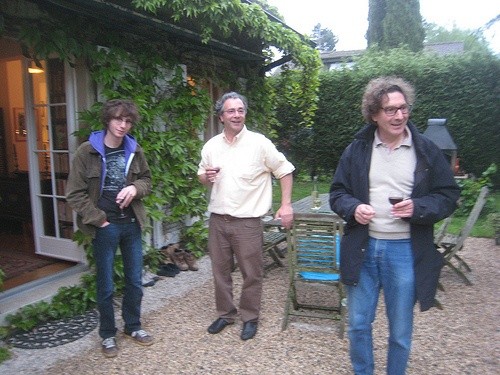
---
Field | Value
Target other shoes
[207,316,235,334]
[241,321,257,339]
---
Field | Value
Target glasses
[223,108,247,116]
[112,116,136,128]
[379,104,413,116]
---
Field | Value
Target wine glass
[116,193,127,218]
[387,190,405,219]
[209,161,220,182]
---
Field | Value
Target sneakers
[123,329,155,346]
[102,336,118,357]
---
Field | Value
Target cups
[310,190,321,211]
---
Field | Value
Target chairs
[230,205,288,277]
[280,219,346,340]
[431,186,488,283]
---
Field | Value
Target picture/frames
[13,107,41,142]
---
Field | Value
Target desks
[288,191,343,264]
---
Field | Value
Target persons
[66,99,153,356]
[329,76,459,375]
[197,92,296,339]
